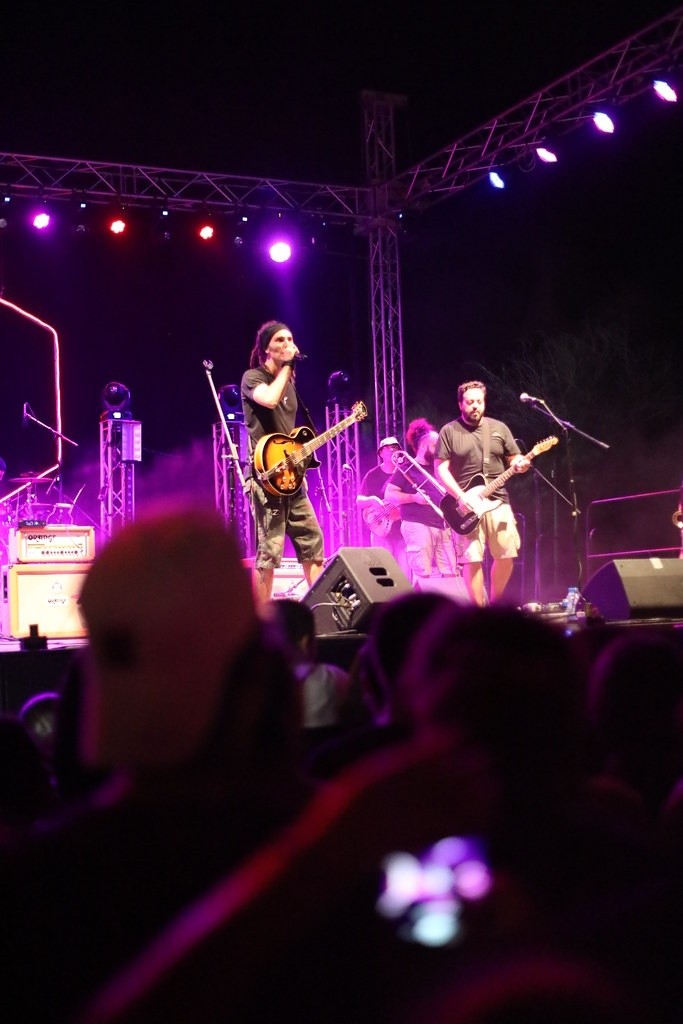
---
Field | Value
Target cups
[528,603,541,612]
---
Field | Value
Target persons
[384,417,460,581]
[434,381,532,606]
[241,321,327,607]
[1,512,683,1024]
[353,437,412,591]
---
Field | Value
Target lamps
[100,381,132,420]
[488,168,514,190]
[328,371,351,404]
[536,140,564,163]
[593,94,624,133]
[217,384,243,421]
[653,66,681,104]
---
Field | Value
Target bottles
[567,588,579,614]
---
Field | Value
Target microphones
[46,476,59,496]
[294,352,306,362]
[520,393,539,403]
[22,404,29,427]
[343,464,352,470]
[274,592,296,597]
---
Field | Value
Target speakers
[0,564,93,640]
[297,546,412,637]
[270,558,327,601]
[575,557,683,622]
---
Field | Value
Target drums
[0,499,72,566]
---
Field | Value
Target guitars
[363,499,402,538]
[253,400,368,497]
[439,435,559,535]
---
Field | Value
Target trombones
[391,450,447,520]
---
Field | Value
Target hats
[377,437,402,459]
[77,512,260,769]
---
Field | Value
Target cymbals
[9,477,53,484]
